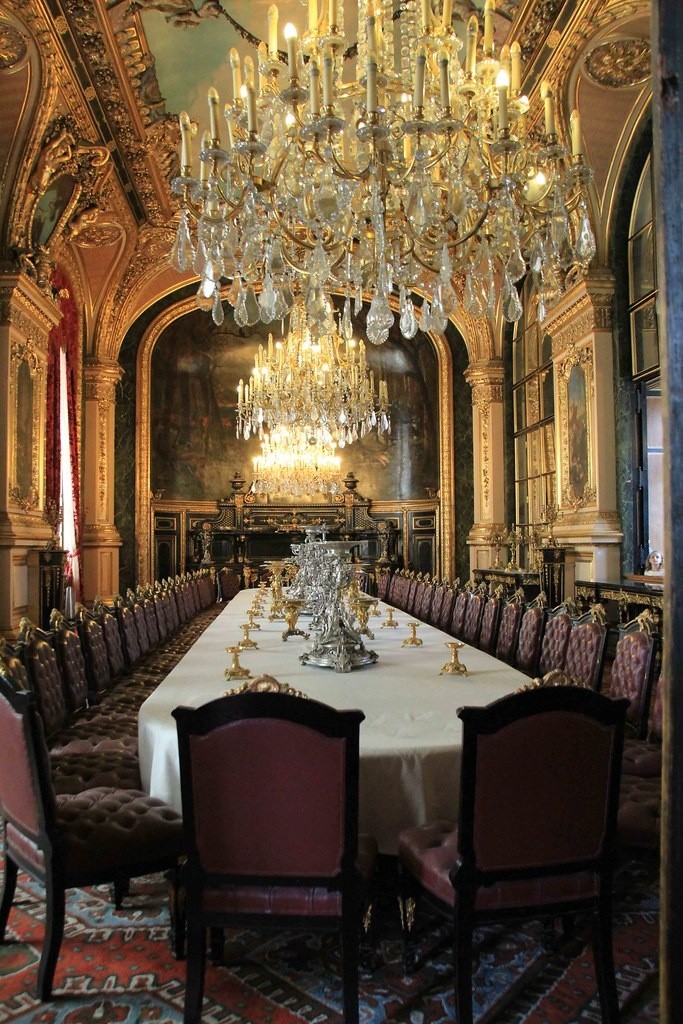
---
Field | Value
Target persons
[644,550,664,586]
[69,203,99,243]
[31,128,72,196]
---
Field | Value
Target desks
[139,587,532,880]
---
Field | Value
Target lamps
[234,291,391,450]
[250,417,343,498]
[170,0,597,346]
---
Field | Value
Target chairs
[0,566,665,1024]
